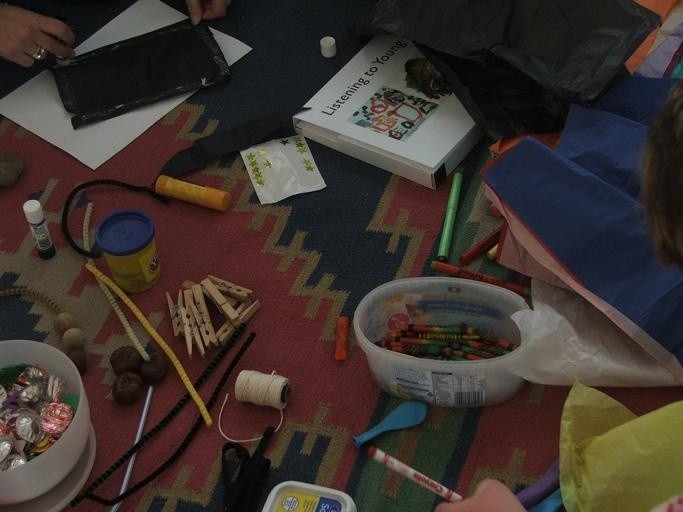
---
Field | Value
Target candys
[0,362,77,471]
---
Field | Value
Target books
[290,32,486,191]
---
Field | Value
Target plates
[0,421,97,512]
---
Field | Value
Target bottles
[92,207,161,297]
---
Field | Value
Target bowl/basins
[0,339,93,506]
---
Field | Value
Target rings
[34,46,47,62]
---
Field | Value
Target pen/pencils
[367,448,465,503]
[430,172,532,298]
[382,324,512,360]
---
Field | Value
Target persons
[0,0,230,72]
[432,76,682,511]
